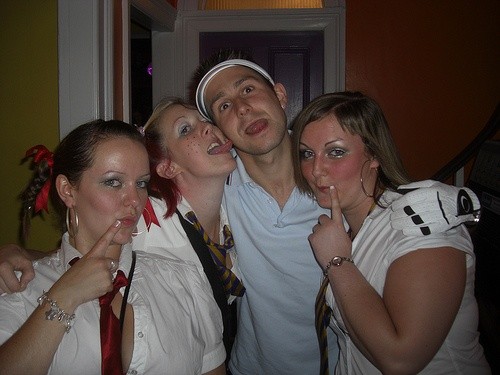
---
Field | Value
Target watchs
[323,256,354,277]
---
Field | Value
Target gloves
[390,179,481,237]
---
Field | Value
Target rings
[109,258,119,272]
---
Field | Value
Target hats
[196,59,275,122]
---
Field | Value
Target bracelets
[37,289,76,334]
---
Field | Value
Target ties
[311,192,384,375]
[69,257,129,375]
[185,211,246,298]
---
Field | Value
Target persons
[0,96,246,375]
[0,119,227,375]
[291,91,491,375]
[185,46,481,375]
